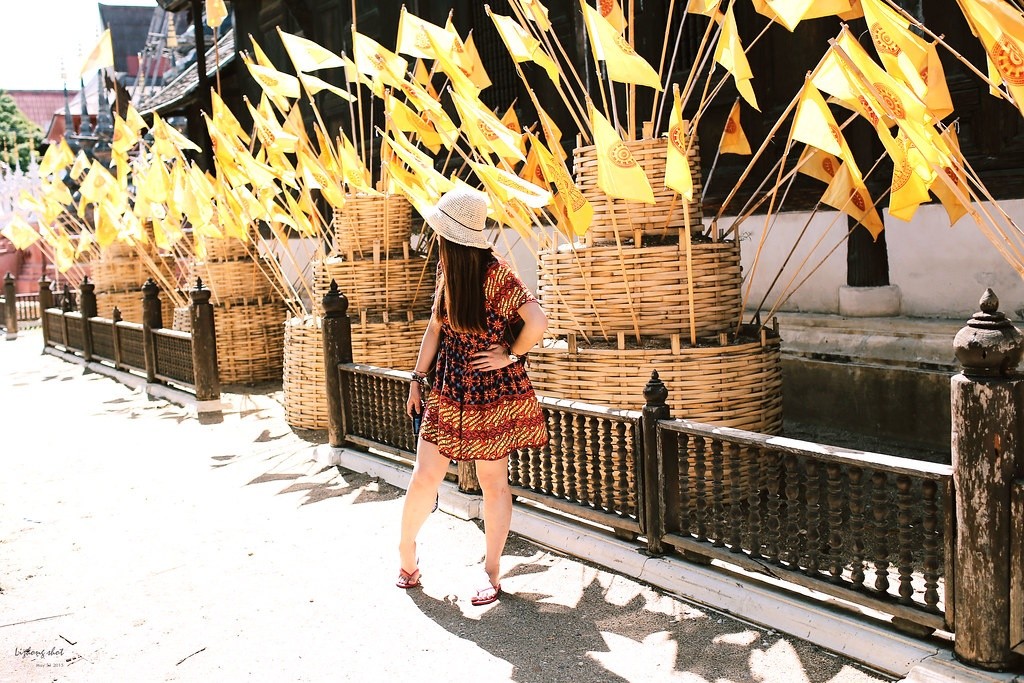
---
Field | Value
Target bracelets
[410,369,427,385]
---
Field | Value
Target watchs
[507,347,528,362]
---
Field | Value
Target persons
[394,184,548,605]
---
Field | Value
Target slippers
[471,566,501,604]
[397,555,420,588]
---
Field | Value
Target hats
[419,188,492,249]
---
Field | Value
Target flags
[0,0,1024,341]
